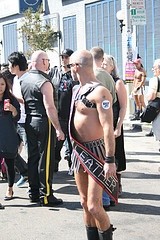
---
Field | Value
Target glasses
[67,64,78,68]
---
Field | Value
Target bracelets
[105,156,115,163]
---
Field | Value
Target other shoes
[4,191,13,200]
[17,176,29,186]
[40,195,63,207]
[146,132,154,136]
[103,205,111,211]
[68,167,73,175]
[110,201,116,206]
[30,193,44,202]
[0,204,4,209]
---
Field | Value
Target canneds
[4,99,10,110]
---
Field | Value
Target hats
[59,49,73,57]
[133,59,141,64]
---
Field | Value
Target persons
[147,59,160,142]
[90,47,116,209]
[131,58,147,121]
[0,52,29,201]
[101,54,126,197]
[68,50,116,240]
[46,48,80,176]
[21,50,64,206]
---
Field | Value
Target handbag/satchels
[140,98,160,123]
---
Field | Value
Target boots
[86,226,99,240]
[131,110,140,120]
[98,224,113,240]
[116,173,122,196]
